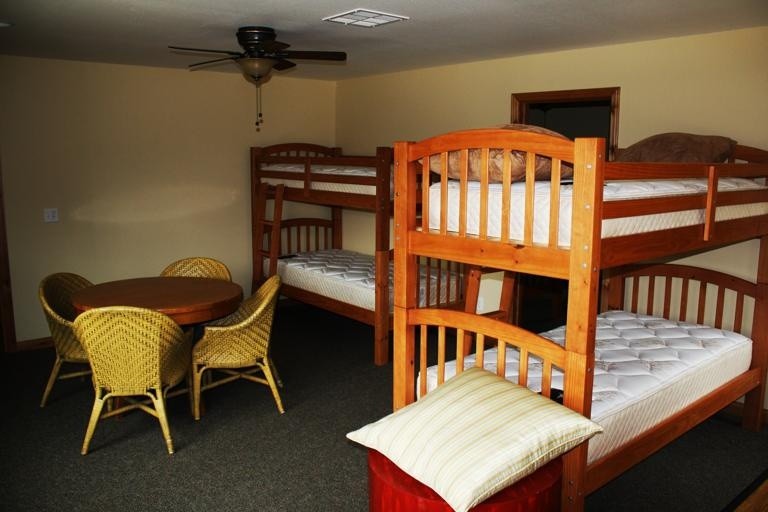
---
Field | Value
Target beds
[397,123,767,511]
[250,142,469,366]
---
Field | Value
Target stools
[368,446,562,512]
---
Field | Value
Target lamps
[236,57,280,133]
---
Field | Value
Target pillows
[609,132,738,163]
[345,365,604,511]
[417,123,574,184]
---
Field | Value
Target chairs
[192,274,286,421]
[72,305,194,455]
[161,257,243,386]
[39,272,114,413]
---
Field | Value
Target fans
[168,25,347,71]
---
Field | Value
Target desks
[69,276,243,420]
